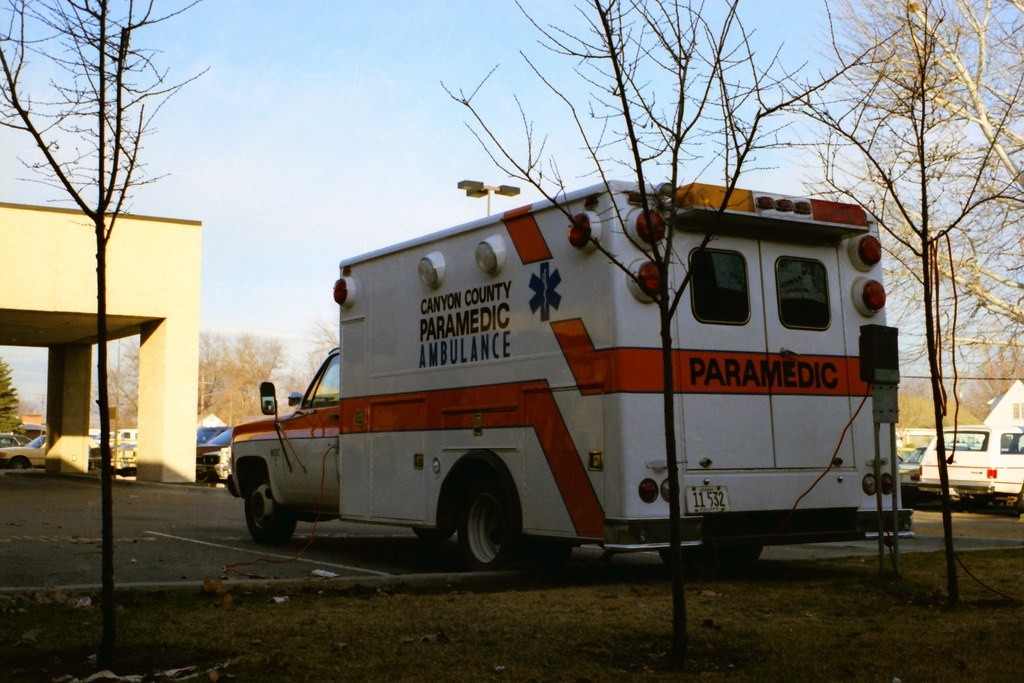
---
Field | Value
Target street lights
[458,179,521,215]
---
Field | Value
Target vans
[916,424,1024,515]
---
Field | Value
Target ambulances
[226,179,916,573]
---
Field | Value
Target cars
[0,424,234,485]
[898,442,981,511]
